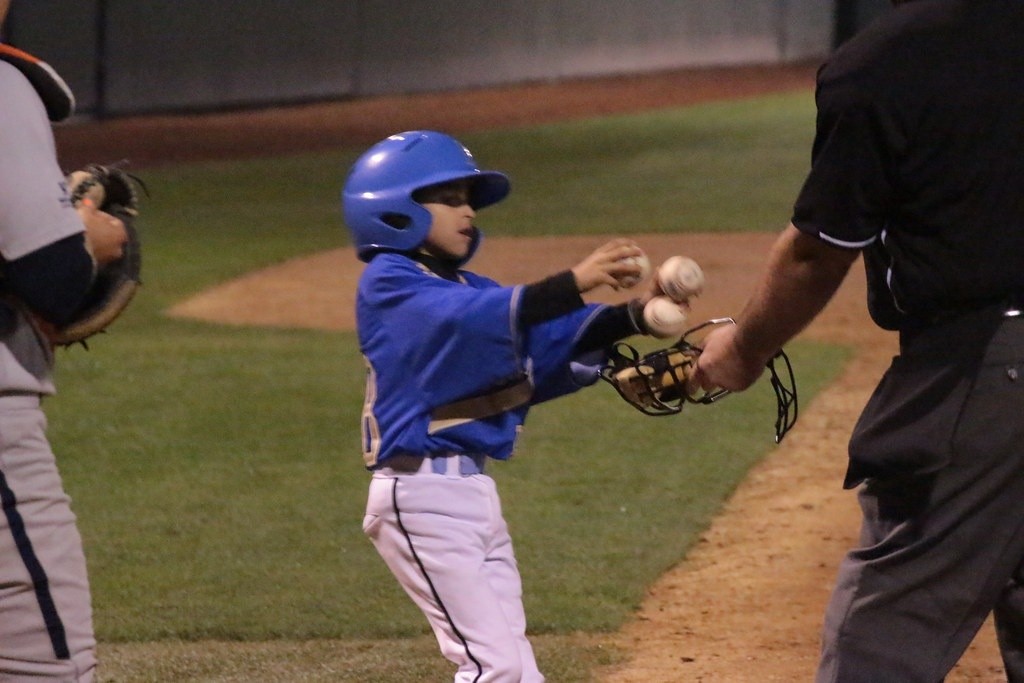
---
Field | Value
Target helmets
[340,129,512,268]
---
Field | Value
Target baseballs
[615,247,648,286]
[643,295,692,339]
[657,255,704,300]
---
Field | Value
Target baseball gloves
[40,160,142,348]
[612,314,740,416]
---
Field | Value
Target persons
[596,0,1024,683]
[338,130,692,683]
[0,0,142,681]
